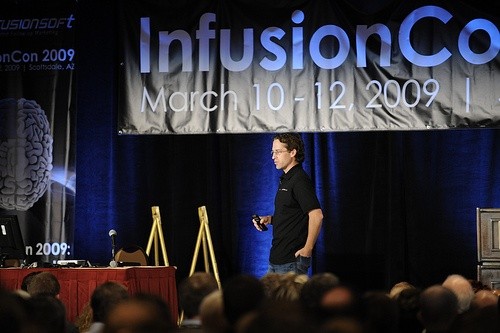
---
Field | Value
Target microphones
[109,261,140,267]
[109,230,117,257]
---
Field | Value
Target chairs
[114,246,150,266]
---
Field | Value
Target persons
[251,131,323,274]
[176,271,218,333]
[81,281,129,333]
[199,271,500,333]
[0,271,81,333]
[104,292,177,333]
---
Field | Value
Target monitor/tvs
[0,215,27,267]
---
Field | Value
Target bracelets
[267,216,269,224]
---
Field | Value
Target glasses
[270,148,289,155]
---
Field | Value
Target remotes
[252,215,268,231]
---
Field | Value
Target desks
[0,264,179,327]
